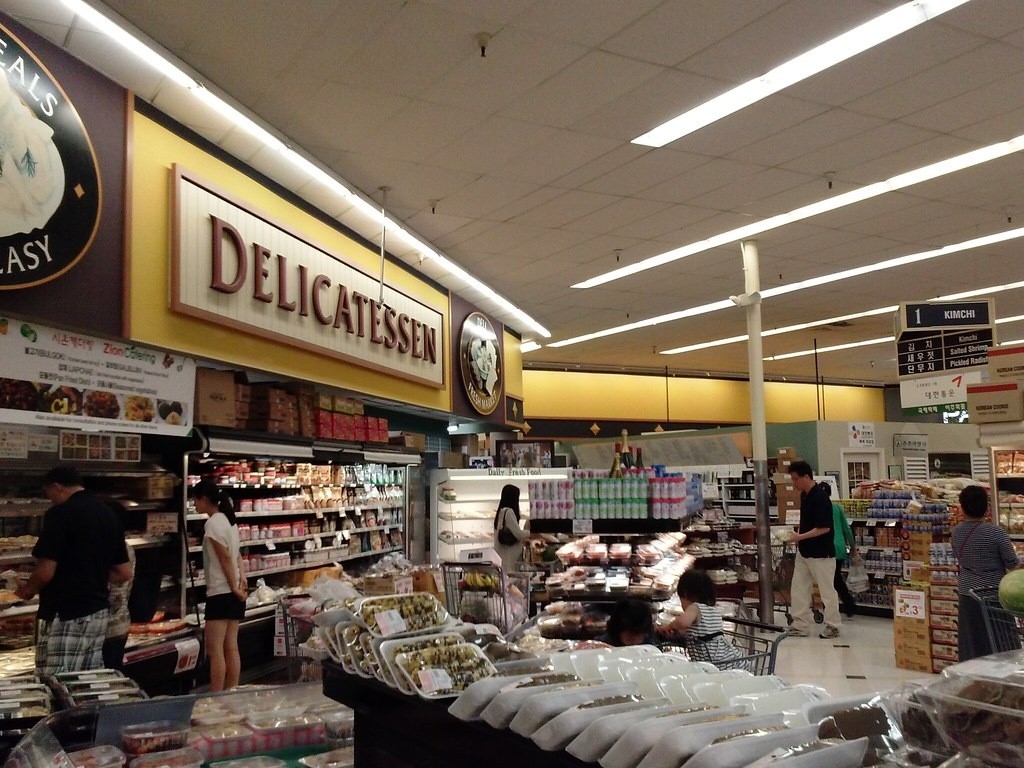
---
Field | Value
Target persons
[784,461,841,638]
[191,481,248,692]
[658,570,749,671]
[493,484,530,570]
[951,485,1021,664]
[819,482,858,620]
[101,502,136,670]
[14,466,133,677]
[598,601,686,648]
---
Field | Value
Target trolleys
[649,616,786,676]
[439,559,535,634]
[744,538,824,626]
[280,593,324,681]
[969,583,1024,654]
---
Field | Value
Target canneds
[234,498,305,573]
[528,478,687,519]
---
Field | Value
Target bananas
[466,569,485,587]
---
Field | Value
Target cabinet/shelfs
[187,425,422,694]
[682,526,760,599]
[430,468,573,567]
[665,463,779,518]
[841,519,902,620]
[989,446,1024,541]
[0,408,204,697]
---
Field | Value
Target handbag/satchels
[498,509,518,546]
[846,552,870,592]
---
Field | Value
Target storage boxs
[274,608,309,657]
[967,343,1024,424]
[875,528,897,547]
[289,567,342,587]
[784,560,824,610]
[364,571,446,605]
[773,448,804,524]
[194,368,426,453]
[893,520,961,674]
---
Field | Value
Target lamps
[60,0,551,340]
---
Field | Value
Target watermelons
[997,569,1024,614]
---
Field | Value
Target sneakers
[786,625,807,636]
[820,625,839,639]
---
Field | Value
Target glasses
[791,476,799,483]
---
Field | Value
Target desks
[529,592,674,617]
[323,659,601,768]
